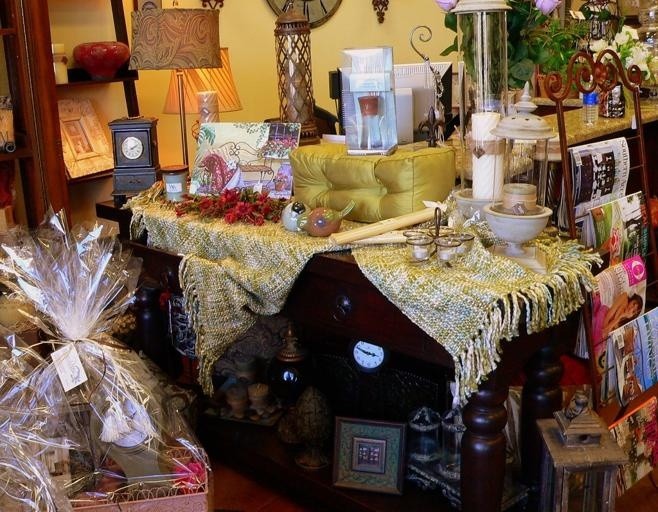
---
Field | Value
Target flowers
[587,29,648,82]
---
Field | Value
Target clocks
[109,121,161,192]
[90,389,175,498]
[353,341,385,371]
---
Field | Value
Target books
[189,122,302,200]
[556,137,658,498]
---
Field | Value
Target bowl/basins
[454,188,502,219]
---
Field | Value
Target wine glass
[483,202,554,258]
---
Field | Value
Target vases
[601,80,624,117]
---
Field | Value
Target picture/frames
[330,417,407,494]
[58,97,114,179]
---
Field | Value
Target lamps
[163,48,241,113]
[127,8,221,184]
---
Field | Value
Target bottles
[490,80,558,213]
[581,75,600,127]
[406,402,465,480]
[160,164,188,203]
[51,43,69,85]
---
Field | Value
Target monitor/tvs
[336,62,453,144]
